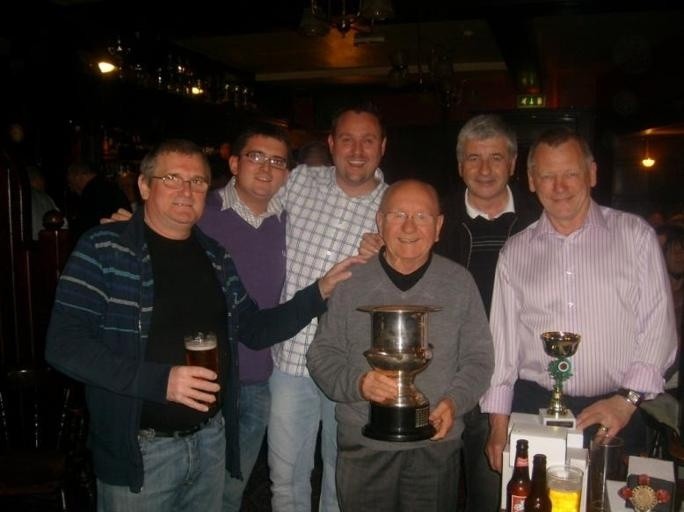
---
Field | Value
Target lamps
[302,0,393,38]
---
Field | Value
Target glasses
[238,150,289,171]
[147,174,211,194]
[379,210,435,227]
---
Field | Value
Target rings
[599,424,608,432]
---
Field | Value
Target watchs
[615,387,642,409]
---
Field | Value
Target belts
[140,418,215,439]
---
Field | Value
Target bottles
[507,440,531,512]
[524,454,552,512]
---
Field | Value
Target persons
[265,105,391,511]
[305,178,494,508]
[24,165,68,241]
[43,135,367,511]
[662,236,684,352]
[62,158,133,222]
[475,128,679,475]
[356,113,530,512]
[97,124,295,512]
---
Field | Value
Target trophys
[356,302,443,443]
[539,329,582,430]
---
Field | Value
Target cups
[184,331,220,408]
[590,436,624,512]
[546,465,584,512]
